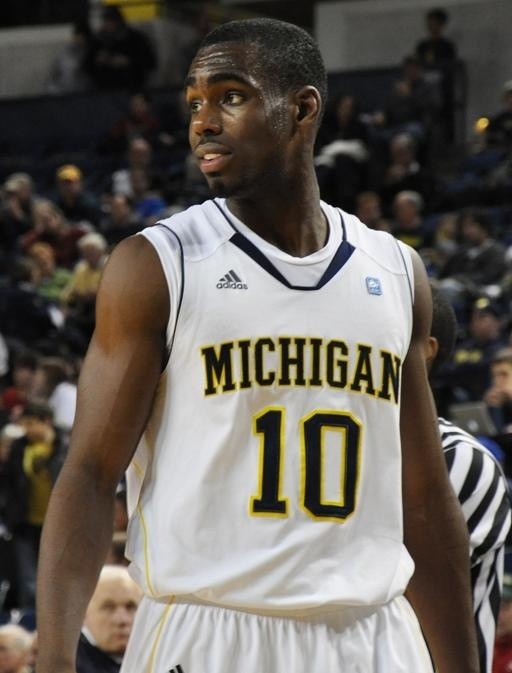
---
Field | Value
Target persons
[0,2,511,672]
